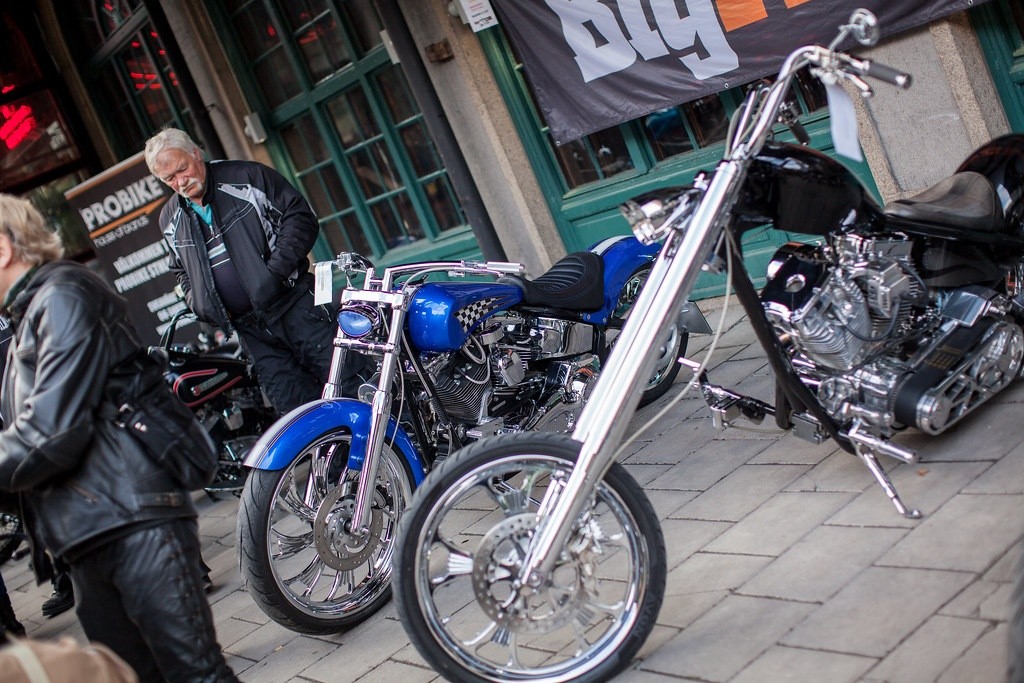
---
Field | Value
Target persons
[0,194,244,683]
[144,129,372,416]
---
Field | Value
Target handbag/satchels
[12,332,218,491]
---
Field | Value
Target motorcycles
[142,303,272,496]
[230,245,692,638]
[389,10,1024,683]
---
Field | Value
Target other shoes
[42,588,73,615]
[3,619,25,636]
[200,575,213,592]
